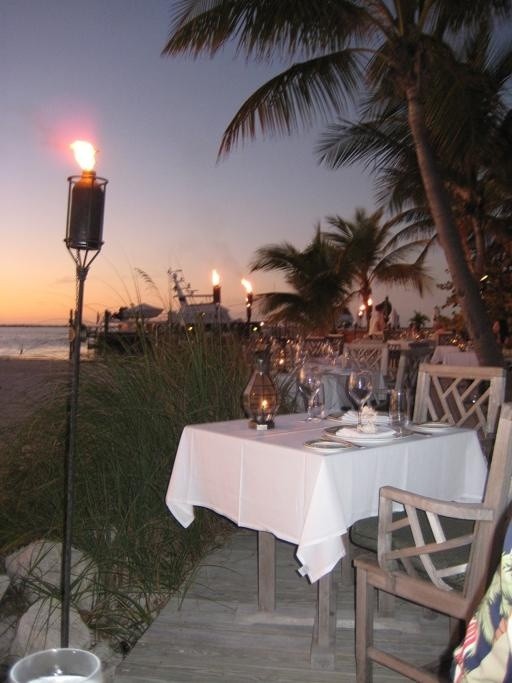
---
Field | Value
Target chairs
[253,333,507,462]
[350,403,512,683]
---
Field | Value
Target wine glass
[295,367,322,422]
[322,344,338,373]
[347,371,374,433]
[389,389,411,437]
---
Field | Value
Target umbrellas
[122,302,163,319]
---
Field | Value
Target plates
[417,420,456,430]
[323,424,403,444]
[303,440,352,451]
